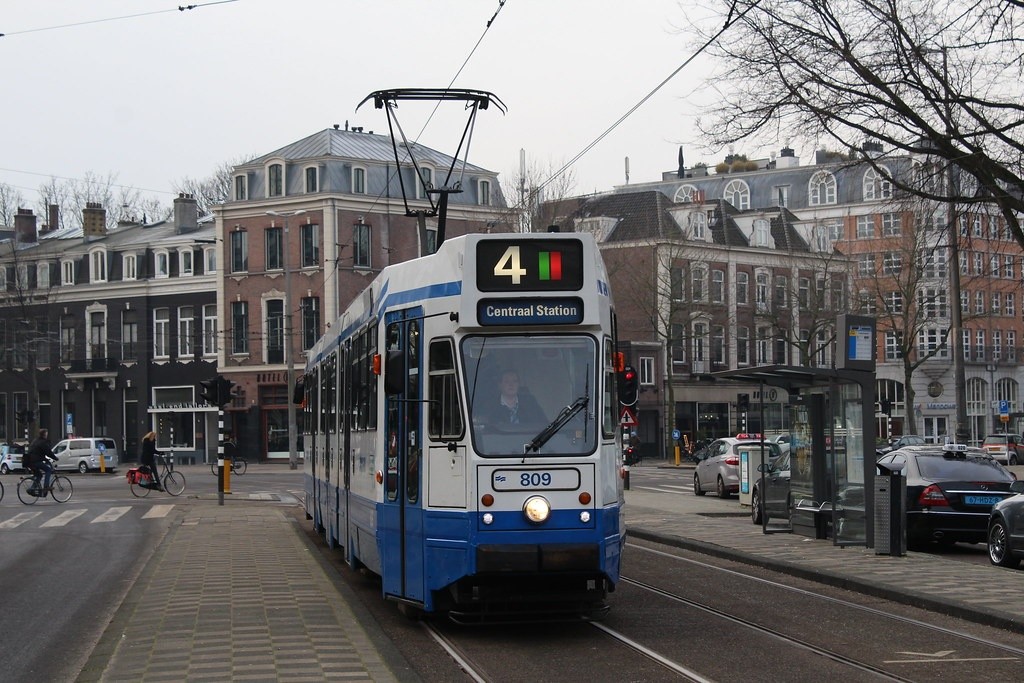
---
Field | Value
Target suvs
[875,434,926,459]
[981,433,1024,466]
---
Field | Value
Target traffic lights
[14,411,27,423]
[200,378,218,405]
[623,367,637,400]
[222,378,238,404]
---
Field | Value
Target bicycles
[212,445,247,476]
[129,454,187,499]
[17,459,73,506]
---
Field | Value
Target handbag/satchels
[22,454,31,467]
[127,467,140,484]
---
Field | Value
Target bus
[291,86,629,628]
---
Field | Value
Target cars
[0,444,31,474]
[751,447,883,525]
[839,444,1018,552]
[986,480,1024,567]
[693,432,782,499]
[765,432,808,457]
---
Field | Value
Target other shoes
[45,487,55,491]
[157,486,166,492]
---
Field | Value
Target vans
[39,437,118,473]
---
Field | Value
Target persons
[139,432,165,492]
[27,429,59,491]
[223,437,236,465]
[481,370,547,423]
[687,437,711,461]
[630,432,640,446]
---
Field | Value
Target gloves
[159,451,165,455]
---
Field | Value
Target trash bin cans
[874,462,906,556]
